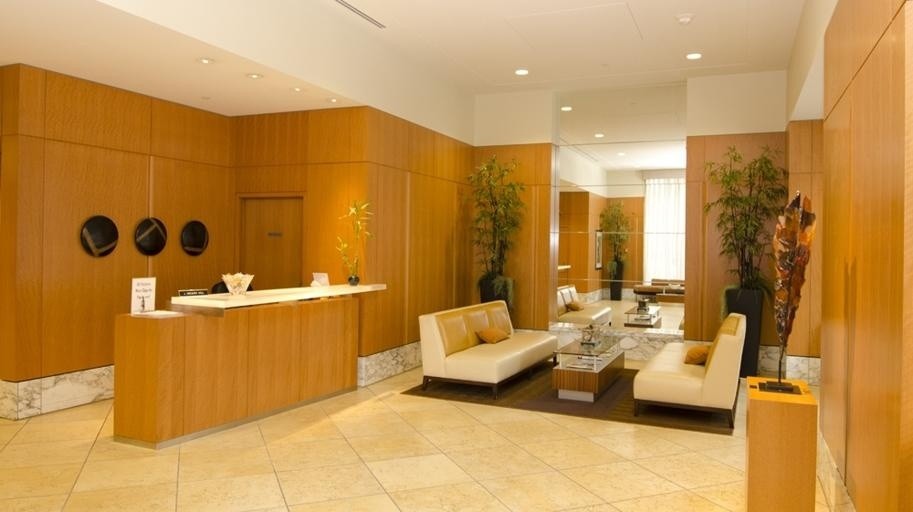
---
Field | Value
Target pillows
[685,344,709,365]
[475,328,510,343]
[566,300,583,310]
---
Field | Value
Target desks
[744,376,818,512]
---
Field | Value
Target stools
[633,286,663,302]
[665,286,685,295]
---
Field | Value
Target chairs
[632,313,747,428]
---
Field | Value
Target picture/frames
[595,230,603,271]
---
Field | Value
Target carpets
[401,361,741,436]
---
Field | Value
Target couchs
[417,299,558,399]
[556,284,613,327]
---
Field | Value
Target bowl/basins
[221,272,254,297]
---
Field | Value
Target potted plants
[600,200,632,300]
[465,155,526,310]
[336,202,375,286]
[704,141,787,378]
